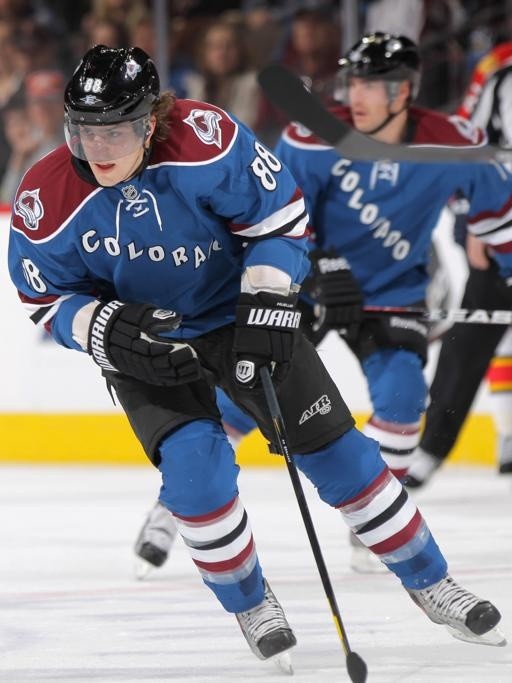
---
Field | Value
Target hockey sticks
[259,364,369,682]
[256,60,510,161]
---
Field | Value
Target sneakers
[132,499,179,570]
[230,577,298,661]
[406,572,502,637]
[395,447,444,489]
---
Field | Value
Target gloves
[86,300,204,392]
[227,290,295,396]
[309,250,370,345]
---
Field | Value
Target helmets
[341,30,423,104]
[60,40,160,127]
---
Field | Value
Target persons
[5,40,502,661]
[131,28,512,571]
[398,38,511,489]
